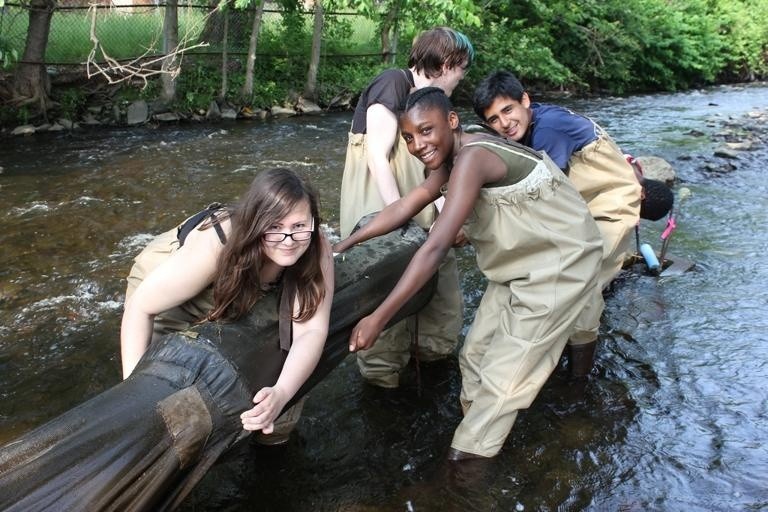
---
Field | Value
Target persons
[331,86,604,459]
[121,167,334,446]
[339,26,474,389]
[617,147,673,221]
[472,71,642,377]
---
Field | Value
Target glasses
[261,216,316,242]
